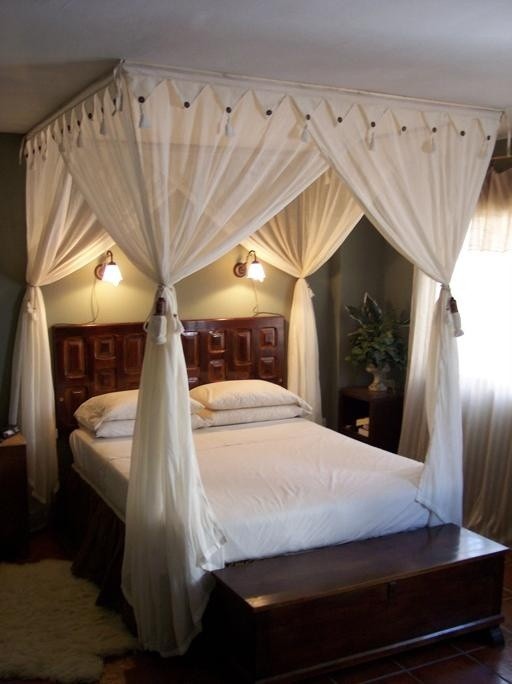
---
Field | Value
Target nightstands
[332,382,405,454]
[1,426,29,562]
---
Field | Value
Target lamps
[231,248,267,281]
[92,248,124,288]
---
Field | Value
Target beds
[48,314,465,646]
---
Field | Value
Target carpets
[0,547,138,681]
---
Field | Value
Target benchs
[203,519,510,679]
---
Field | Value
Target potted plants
[338,287,414,393]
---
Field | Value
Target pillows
[187,378,316,413]
[194,401,313,427]
[73,410,214,438]
[69,384,206,431]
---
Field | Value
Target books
[355,416,369,437]
